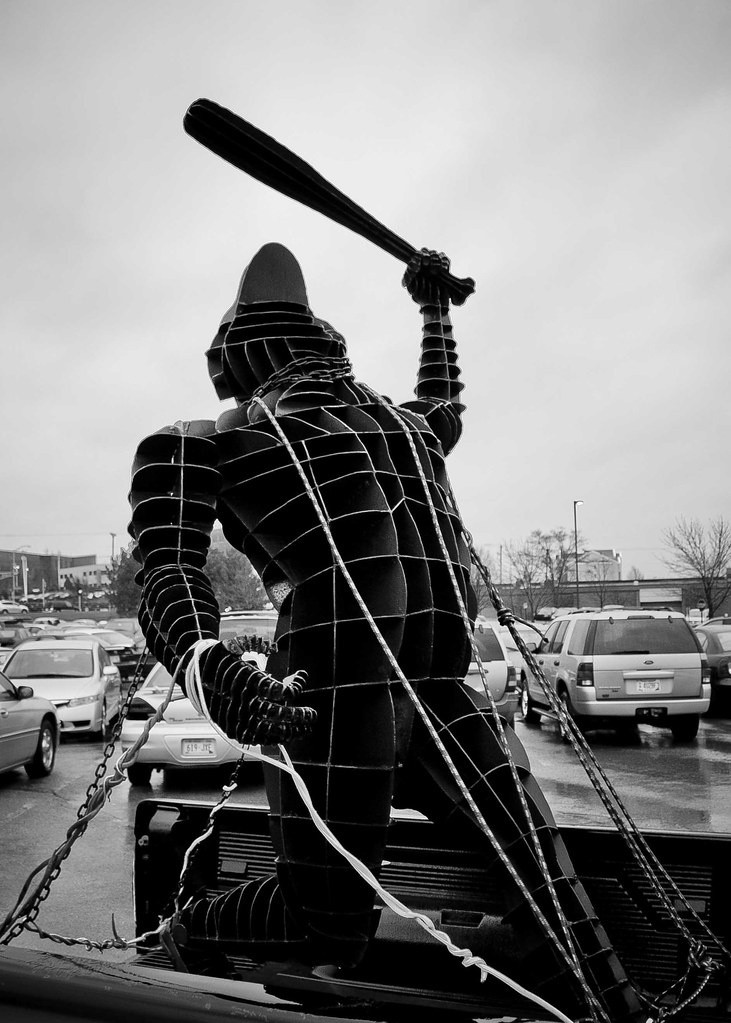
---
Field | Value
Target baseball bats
[180,97,476,307]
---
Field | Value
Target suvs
[519,607,712,745]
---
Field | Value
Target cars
[0,673,60,782]
[695,616,731,628]
[462,625,519,735]
[5,640,122,742]
[0,606,626,671]
[690,625,731,699]
[0,600,30,616]
[119,657,265,787]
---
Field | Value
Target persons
[127,243,669,1023]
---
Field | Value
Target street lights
[574,500,584,612]
[12,544,32,596]
